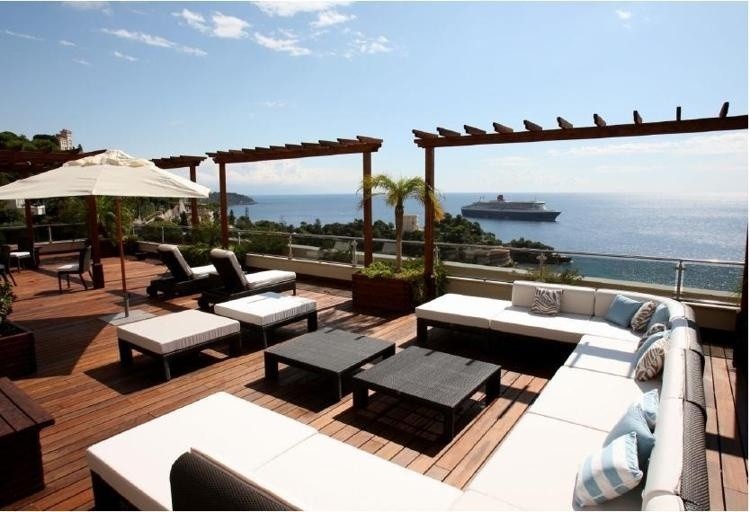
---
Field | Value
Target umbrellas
[0,150,211,318]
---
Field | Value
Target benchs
[0,377,55,508]
[32,237,89,270]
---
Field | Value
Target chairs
[9,234,36,272]
[57,240,93,291]
[0,246,17,287]
[146,244,241,298]
[196,247,297,312]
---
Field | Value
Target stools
[87,390,318,512]
[117,309,242,381]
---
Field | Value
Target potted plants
[0,278,38,379]
[350,172,450,316]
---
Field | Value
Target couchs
[169,281,708,512]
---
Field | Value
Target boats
[460,193,562,222]
